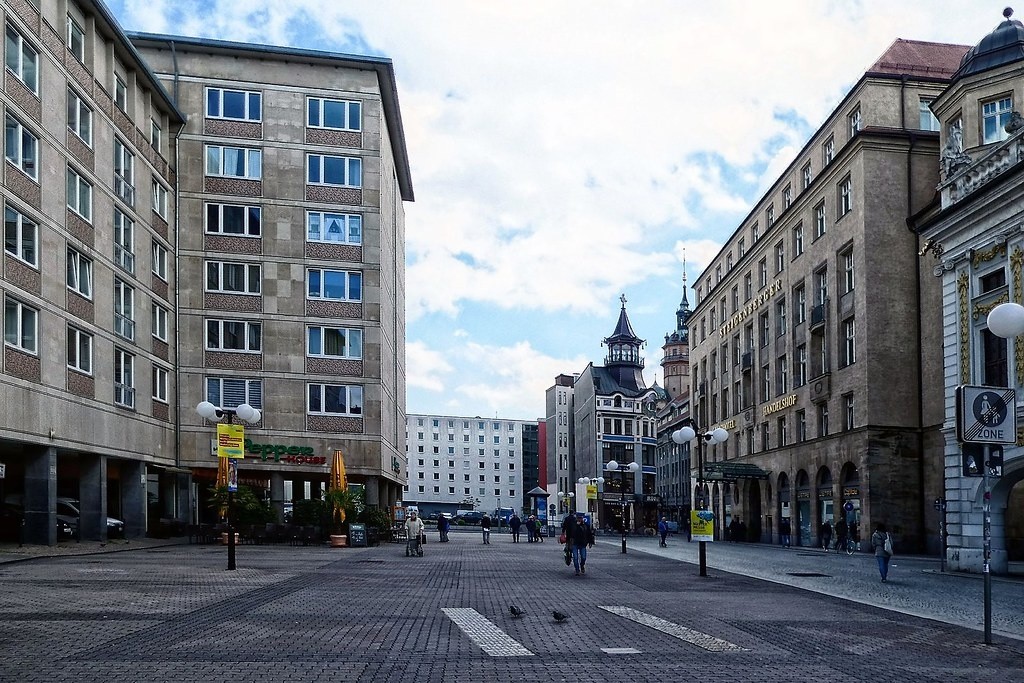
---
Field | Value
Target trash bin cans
[549,525,556,537]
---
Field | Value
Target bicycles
[836,532,856,555]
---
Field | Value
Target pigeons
[552,610,567,623]
[509,605,527,618]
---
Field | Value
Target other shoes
[581,566,585,573]
[487,541,490,544]
[881,578,886,583]
[576,569,580,574]
[439,538,449,542]
[483,541,486,544]
[527,538,544,543]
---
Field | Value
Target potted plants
[293,485,390,546]
[207,482,283,545]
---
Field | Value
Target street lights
[195,401,261,570]
[607,461,638,555]
[558,492,574,513]
[672,428,729,578]
[579,477,605,535]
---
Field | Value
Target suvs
[453,511,483,525]
[25,497,125,536]
[493,507,514,525]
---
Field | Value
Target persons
[481,514,490,544]
[560,510,576,551]
[833,517,848,552]
[526,514,543,543]
[510,513,521,544]
[850,520,857,546]
[658,517,668,547]
[729,515,740,543]
[436,513,450,542]
[820,518,832,552]
[567,515,594,575]
[872,523,891,583]
[405,511,424,557]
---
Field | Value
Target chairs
[186,523,282,545]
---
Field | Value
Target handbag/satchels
[483,527,490,531]
[884,539,893,555]
[564,551,572,566]
[418,534,427,544]
[560,533,566,543]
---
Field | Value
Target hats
[529,516,533,518]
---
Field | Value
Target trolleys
[406,534,423,557]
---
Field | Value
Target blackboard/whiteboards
[348,522,367,546]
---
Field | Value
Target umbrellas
[215,457,228,518]
[329,450,347,534]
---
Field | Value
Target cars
[5,502,72,541]
